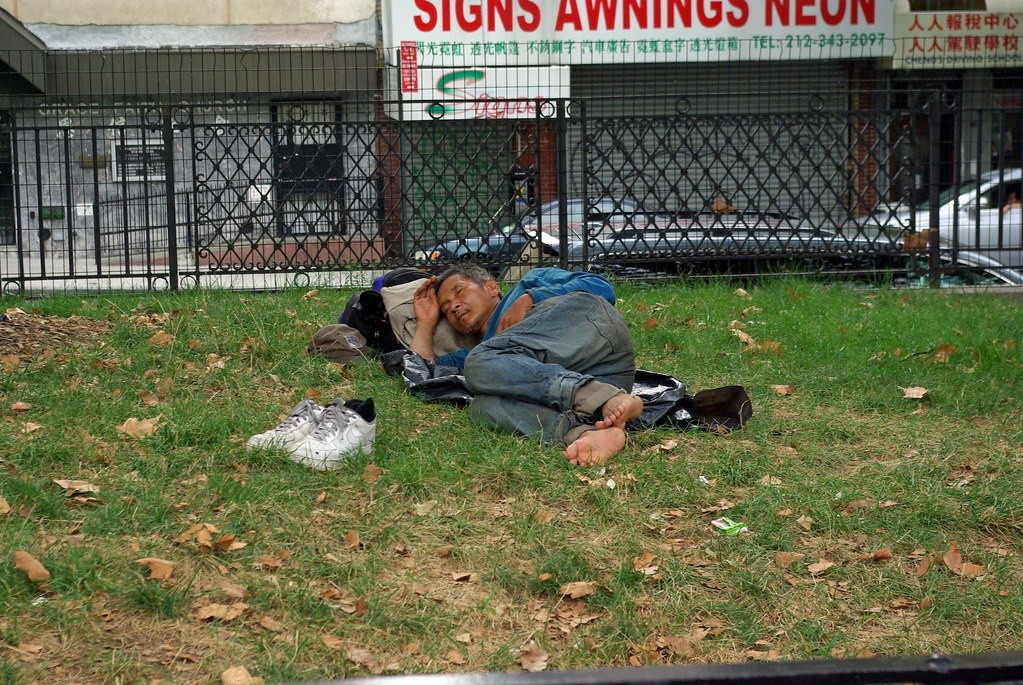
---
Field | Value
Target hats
[307,323,371,364]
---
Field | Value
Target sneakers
[245,398,345,459]
[287,397,378,472]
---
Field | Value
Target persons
[409,263,643,466]
[1003,190,1022,213]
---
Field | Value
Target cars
[853,167,1023,273]
[414,193,1022,285]
